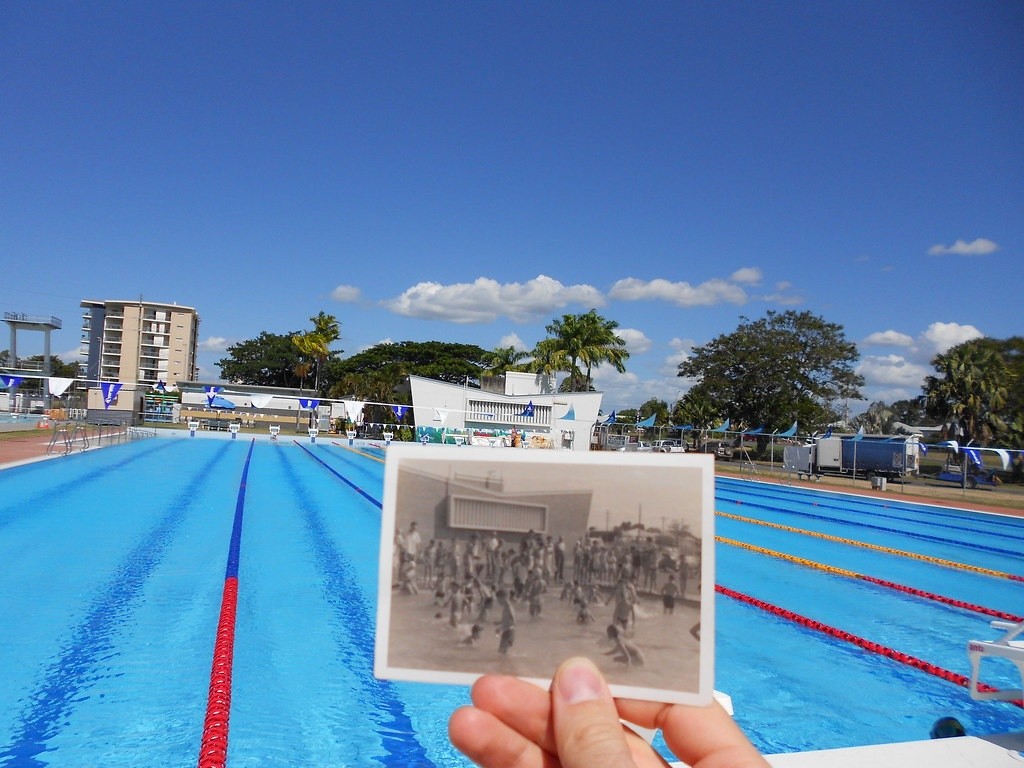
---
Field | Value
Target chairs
[741,461,759,482]
[780,461,800,488]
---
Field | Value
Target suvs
[698,442,734,461]
[651,440,675,452]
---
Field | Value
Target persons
[448,656,771,768]
[930,717,966,739]
[392,520,700,666]
[511,425,517,447]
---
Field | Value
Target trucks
[799,437,919,481]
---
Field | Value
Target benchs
[206,420,231,431]
